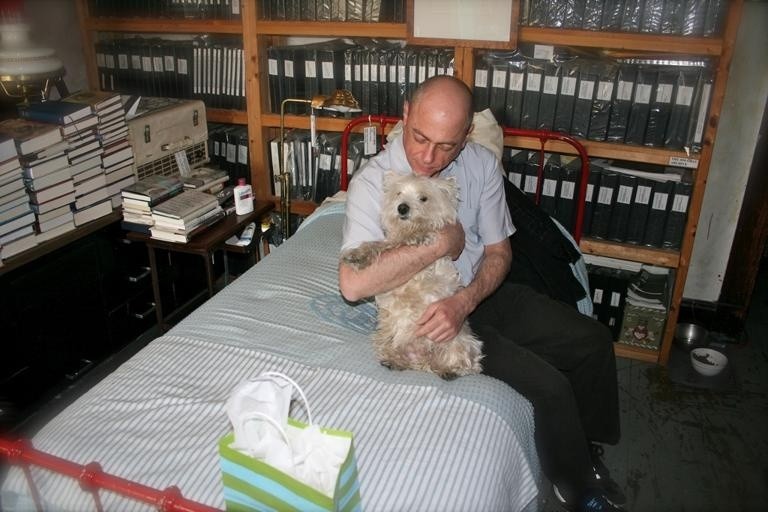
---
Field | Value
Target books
[1,87,143,261]
[469,1,725,154]
[503,149,693,339]
[267,131,389,203]
[257,2,454,118]
[86,2,239,167]
[118,163,234,244]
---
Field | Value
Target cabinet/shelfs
[70,3,742,369]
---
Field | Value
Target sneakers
[586,442,628,507]
[552,481,618,512]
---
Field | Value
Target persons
[336,72,629,510]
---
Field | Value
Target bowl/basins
[674,321,712,353]
[691,348,728,376]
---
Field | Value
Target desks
[1,173,223,387]
[118,198,272,318]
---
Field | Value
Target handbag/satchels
[218,371,362,512]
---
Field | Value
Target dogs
[341,169,488,383]
